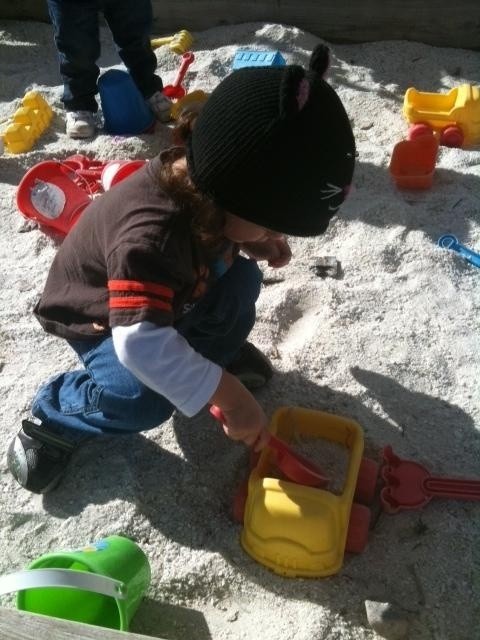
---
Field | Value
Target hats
[182,63,358,241]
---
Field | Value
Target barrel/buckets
[0,535,151,633]
[15,158,147,234]
[96,69,156,135]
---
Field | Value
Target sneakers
[4,402,74,494]
[231,342,273,382]
[147,87,175,122]
[63,108,98,140]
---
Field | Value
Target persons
[45,1,180,140]
[5,62,359,494]
